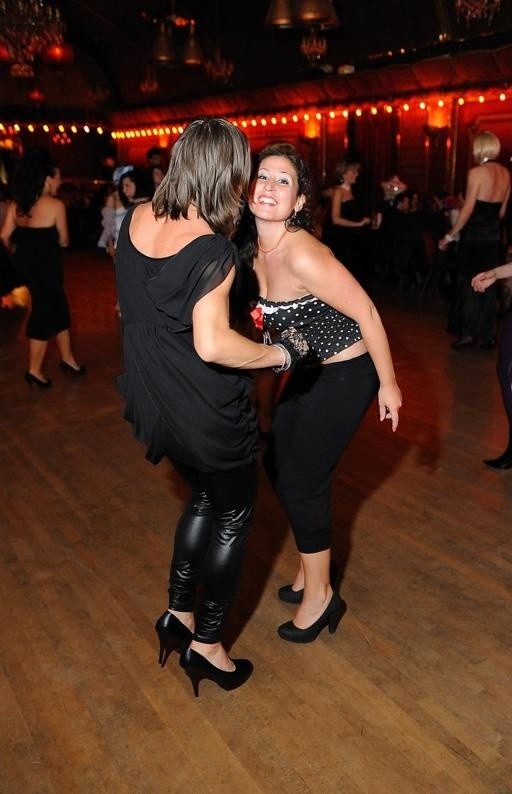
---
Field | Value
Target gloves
[277,325,311,367]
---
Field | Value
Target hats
[113,165,134,186]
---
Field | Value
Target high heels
[278,543,339,604]
[23,370,53,391]
[155,610,194,666]
[277,591,348,643]
[451,335,476,350]
[179,647,254,698]
[480,336,498,351]
[58,361,86,377]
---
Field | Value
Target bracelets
[270,343,286,373]
[277,340,291,371]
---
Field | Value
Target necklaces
[191,202,197,207]
[258,226,288,253]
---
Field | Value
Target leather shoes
[483,447,512,470]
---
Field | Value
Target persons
[1,158,86,391]
[240,141,409,644]
[116,117,307,695]
[97,146,172,264]
[256,366,284,439]
[321,131,511,354]
[465,259,512,469]
